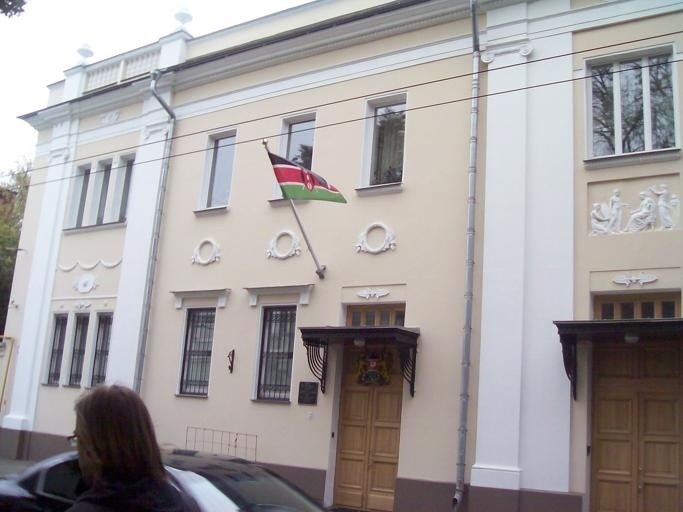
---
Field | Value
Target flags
[267,152,346,204]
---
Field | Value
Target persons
[66,383,193,511]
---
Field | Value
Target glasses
[67,435,78,447]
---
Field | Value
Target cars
[1,445,339,512]
